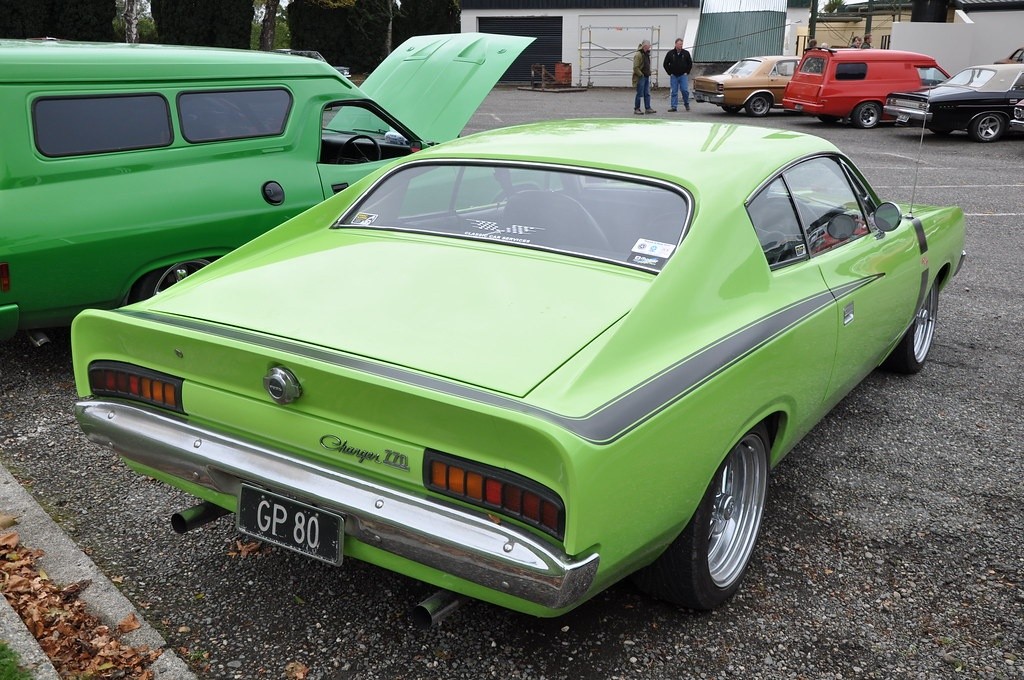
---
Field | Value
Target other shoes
[668,109,677,112]
[686,107,690,110]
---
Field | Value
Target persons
[860,34,873,49]
[664,37,693,113]
[632,40,656,115]
[808,40,829,50]
[850,35,862,49]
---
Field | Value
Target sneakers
[634,108,644,114]
[645,107,657,113]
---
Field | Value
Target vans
[782,46,959,129]
[0,30,540,339]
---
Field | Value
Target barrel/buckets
[555,63,572,86]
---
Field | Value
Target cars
[883,64,1024,141]
[69,116,961,629]
[692,56,818,118]
[993,48,1024,64]
